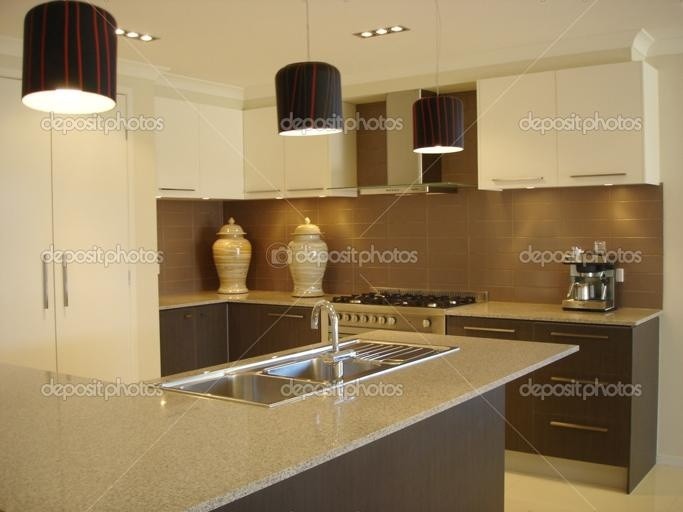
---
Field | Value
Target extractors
[356,89,477,197]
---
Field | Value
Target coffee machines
[561,249,618,313]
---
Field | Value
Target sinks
[160,371,331,407]
[265,337,460,382]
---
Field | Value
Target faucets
[310,299,355,363]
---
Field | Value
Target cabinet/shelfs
[158,288,351,375]
[244,103,358,199]
[0,76,162,383]
[477,71,556,192]
[556,59,661,187]
[446,299,662,490]
[157,91,244,202]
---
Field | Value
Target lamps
[19,1,117,117]
[352,24,410,39]
[115,28,159,43]
[410,1,464,155]
[274,0,343,138]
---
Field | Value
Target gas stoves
[328,287,487,336]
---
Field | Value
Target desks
[0,327,580,511]
